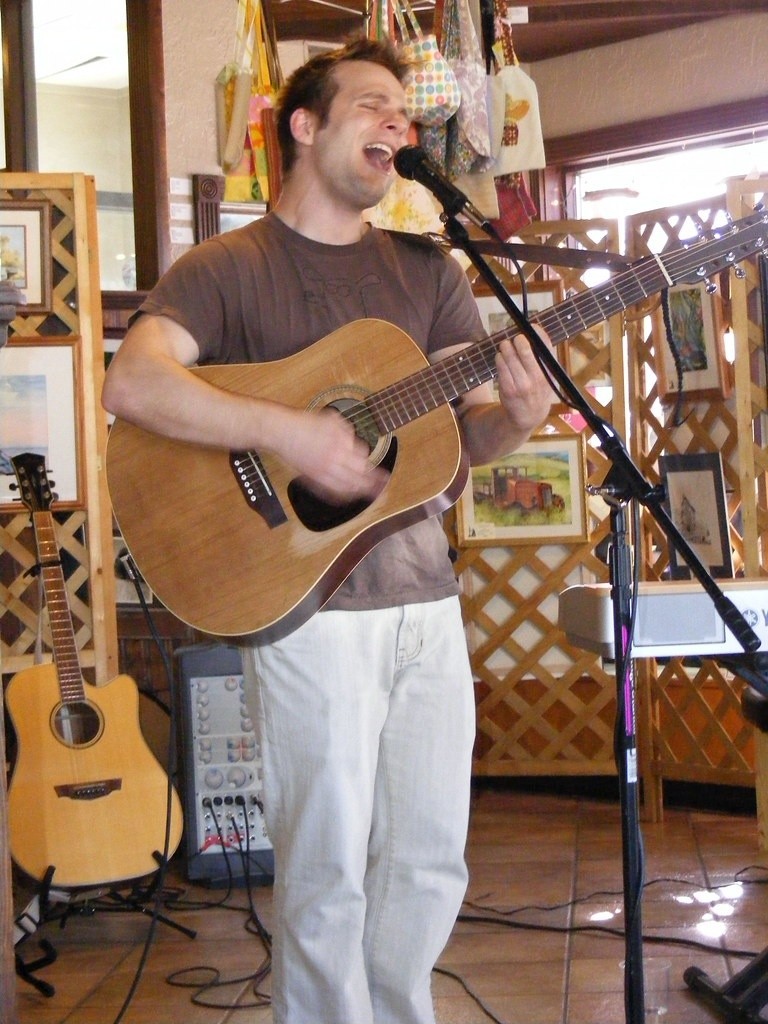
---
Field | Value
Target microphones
[395,143,494,234]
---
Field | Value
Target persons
[100,35,549,1022]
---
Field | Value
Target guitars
[104,197,768,650]
[1,451,186,890]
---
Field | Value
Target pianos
[556,574,768,661]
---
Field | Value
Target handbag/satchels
[364,0,547,239]
[215,1,288,203]
